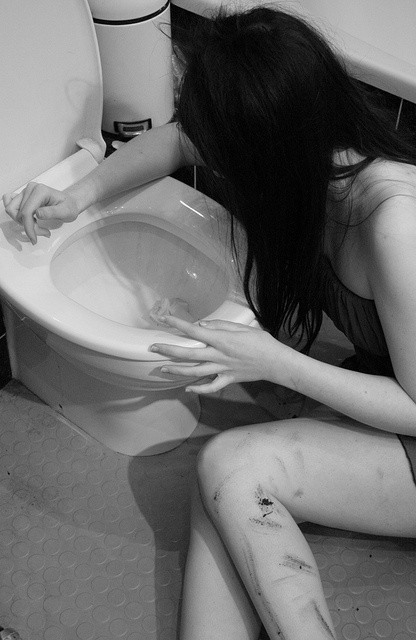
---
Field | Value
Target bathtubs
[171,0,415,191]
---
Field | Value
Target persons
[1,4,415,639]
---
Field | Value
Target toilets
[0,0,259,457]
